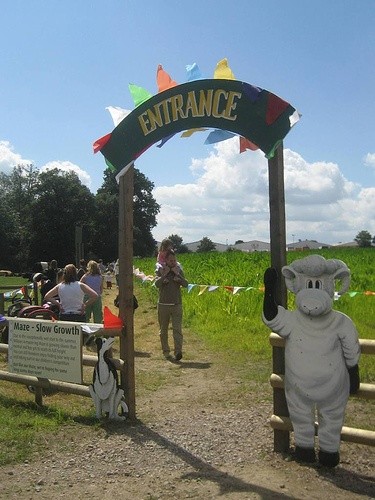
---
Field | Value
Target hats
[32,272,46,281]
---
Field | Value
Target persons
[154,238,188,360]
[33,258,138,346]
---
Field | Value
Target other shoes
[175,351,182,361]
[173,277,179,281]
[163,279,169,283]
[163,350,171,356]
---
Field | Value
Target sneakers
[85,334,95,345]
